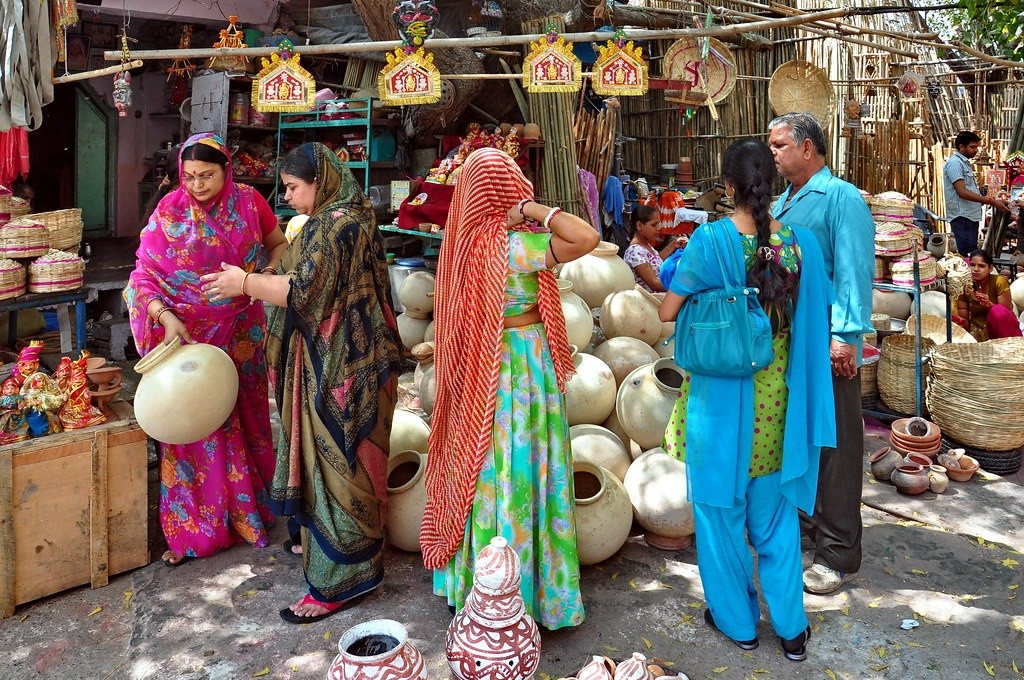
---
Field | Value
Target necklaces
[977,279,991,289]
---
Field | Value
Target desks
[0,291,89,361]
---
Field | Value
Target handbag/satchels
[663,217,775,377]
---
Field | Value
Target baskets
[0,184,88,301]
[854,187,1024,476]
[662,37,736,104]
[768,60,837,130]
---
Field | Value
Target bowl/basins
[937,453,980,482]
[890,417,941,460]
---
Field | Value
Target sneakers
[802,562,860,594]
[801,530,816,550]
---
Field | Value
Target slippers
[161,551,194,566]
[279,591,365,624]
[786,624,811,660]
[705,608,759,650]
[283,538,304,556]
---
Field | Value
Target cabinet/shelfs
[138,68,401,219]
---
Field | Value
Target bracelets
[261,266,278,274]
[241,272,251,296]
[153,305,174,326]
[517,198,533,219]
[544,206,563,229]
[665,246,670,253]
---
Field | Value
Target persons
[623,206,688,294]
[954,249,1023,342]
[200,142,417,627]
[122,131,290,567]
[1015,199,1024,207]
[0,340,107,445]
[420,147,601,632]
[659,136,838,660]
[943,131,1011,258]
[766,113,876,593]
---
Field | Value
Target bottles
[926,234,945,259]
[229,93,249,124]
[444,536,541,680]
[576,653,688,680]
[133,336,239,445]
[868,446,950,495]
[325,618,431,680]
[383,240,697,567]
